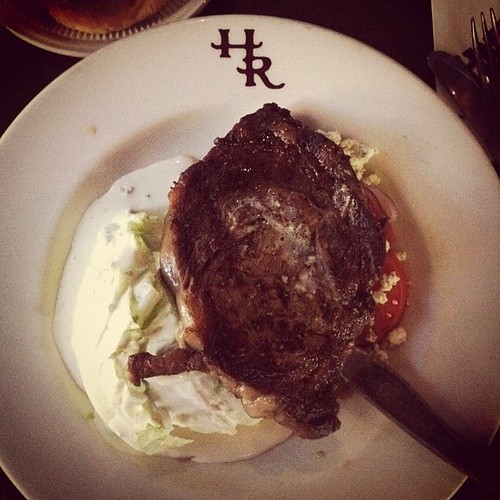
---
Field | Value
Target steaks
[127,102,387,439]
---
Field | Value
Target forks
[469,7,500,98]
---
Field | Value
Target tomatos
[375,251,408,338]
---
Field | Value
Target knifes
[427,49,500,180]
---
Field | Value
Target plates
[0,0,204,59]
[0,16,500,500]
